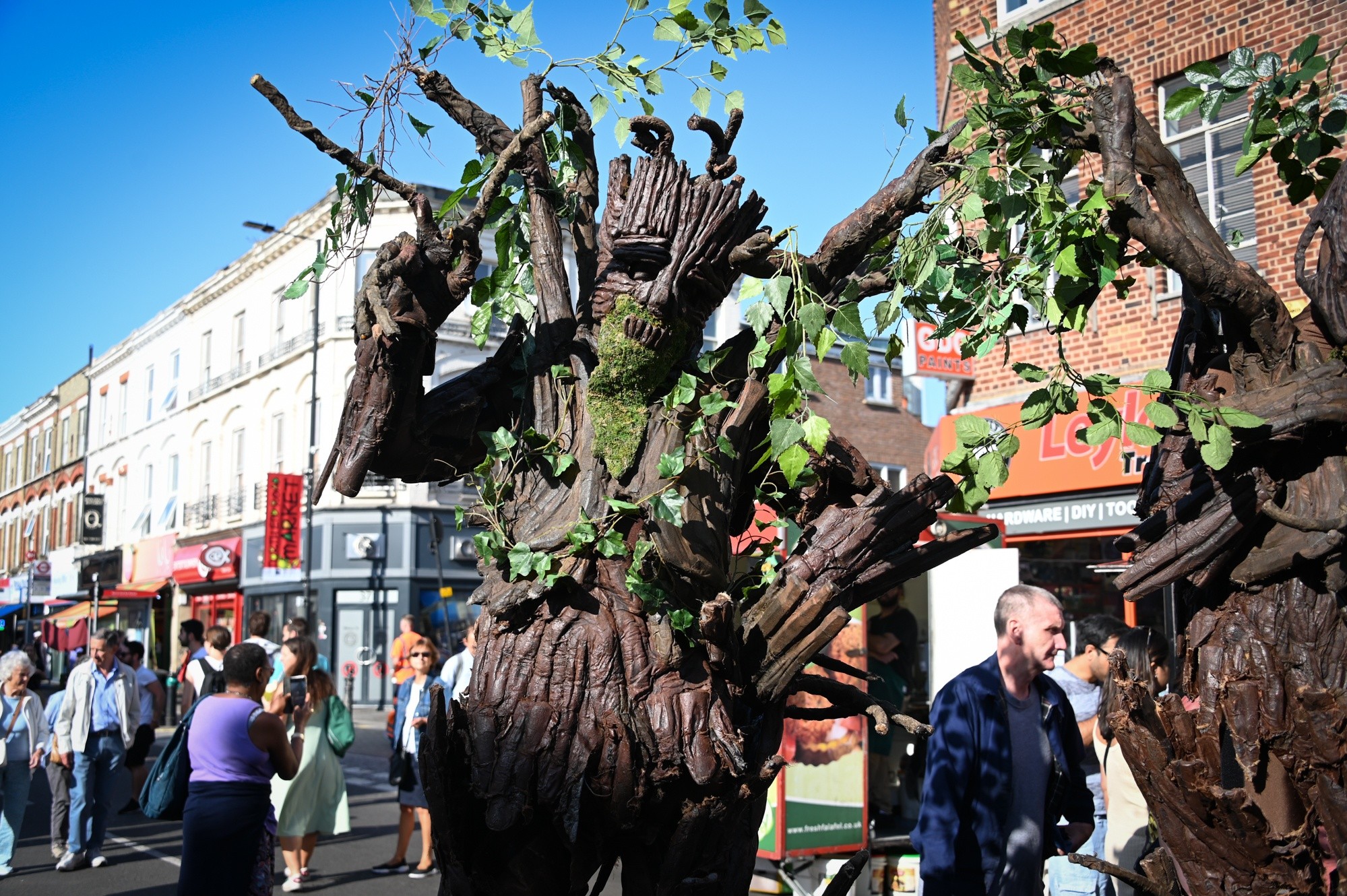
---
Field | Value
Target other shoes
[372,857,409,873]
[50,843,66,858]
[408,860,438,878]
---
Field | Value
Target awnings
[0,601,23,617]
[45,599,119,629]
[117,581,168,593]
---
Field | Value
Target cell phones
[290,674,307,709]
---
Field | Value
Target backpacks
[197,658,228,696]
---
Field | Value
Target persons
[909,585,1095,896]
[11,629,46,692]
[0,650,50,876]
[40,627,165,871]
[372,614,478,880]
[865,583,918,840]
[177,610,349,896]
[1046,614,1201,896]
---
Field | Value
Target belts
[88,730,111,737]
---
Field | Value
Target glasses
[120,652,130,658]
[255,665,274,678]
[1134,624,1157,646]
[411,652,430,656]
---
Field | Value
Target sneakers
[282,874,303,892]
[85,847,109,867]
[284,866,311,880]
[55,850,85,872]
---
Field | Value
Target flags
[262,474,304,585]
[82,493,105,545]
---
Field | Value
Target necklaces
[225,690,253,700]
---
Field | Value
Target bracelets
[291,732,304,742]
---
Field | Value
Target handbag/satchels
[0,737,8,767]
[138,691,214,821]
[325,695,356,758]
[388,749,412,785]
